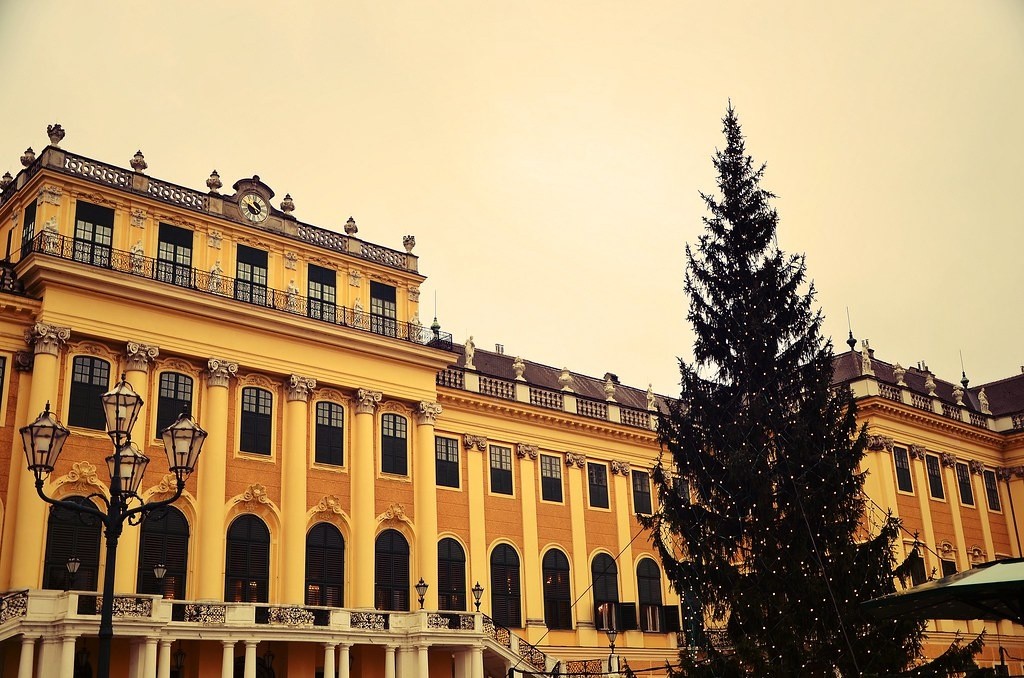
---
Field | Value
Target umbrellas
[864,557,1024,621]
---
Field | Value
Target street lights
[18,371,208,678]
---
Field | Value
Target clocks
[237,191,270,223]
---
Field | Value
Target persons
[408,310,420,341]
[131,239,144,274]
[861,338,872,371]
[977,388,989,405]
[211,259,223,292]
[286,278,299,312]
[44,216,59,253]
[353,296,363,327]
[646,384,656,409]
[463,336,476,364]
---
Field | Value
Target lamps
[153,555,168,595]
[415,577,429,610]
[64,551,82,591]
[472,581,485,612]
[605,626,619,654]
